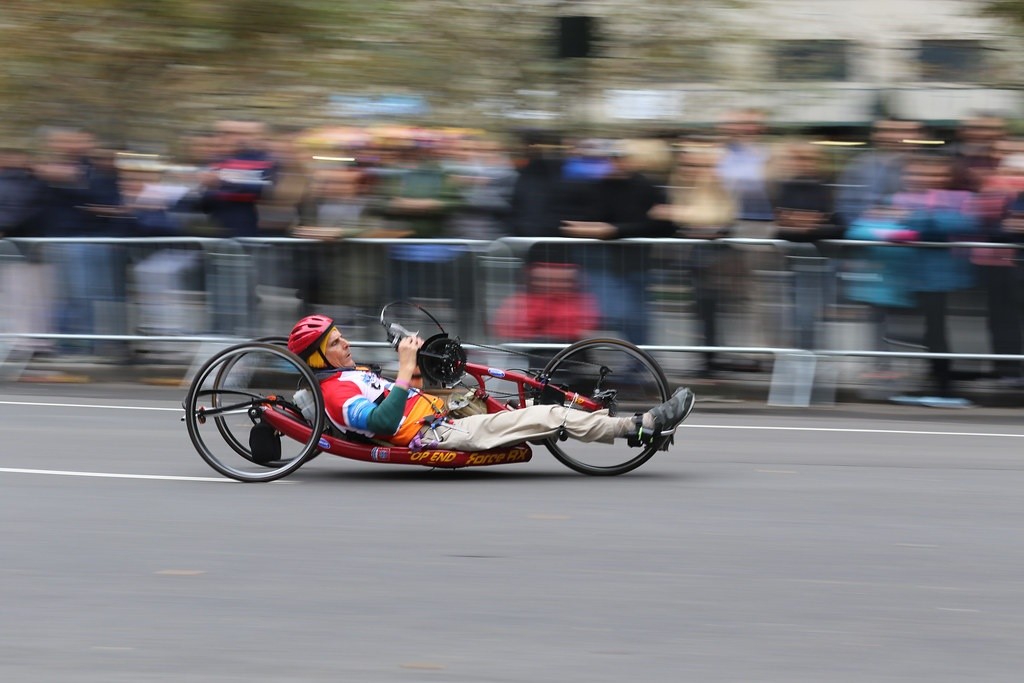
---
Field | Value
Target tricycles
[183,297,674,483]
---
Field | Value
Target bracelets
[396,380,410,387]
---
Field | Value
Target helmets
[288,315,336,359]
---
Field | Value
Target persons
[289,313,696,451]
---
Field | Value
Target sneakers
[638,387,695,450]
